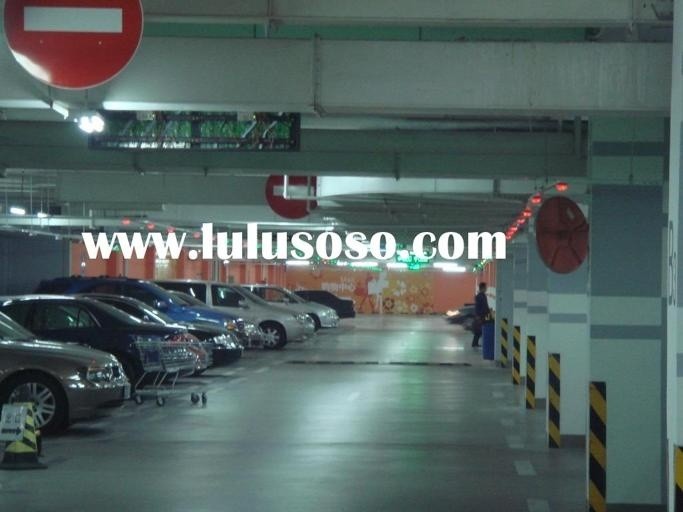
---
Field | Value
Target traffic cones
[1,384,48,469]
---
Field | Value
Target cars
[293,290,355,320]
[0,312,131,434]
[442,302,478,331]
[241,283,339,331]
[0,294,207,387]
[93,295,243,379]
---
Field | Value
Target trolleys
[125,328,208,407]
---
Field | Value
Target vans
[151,279,315,350]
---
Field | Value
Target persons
[472,282,490,346]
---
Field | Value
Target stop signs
[4,0,143,91]
[263,174,317,219]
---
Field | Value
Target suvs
[36,276,262,356]
